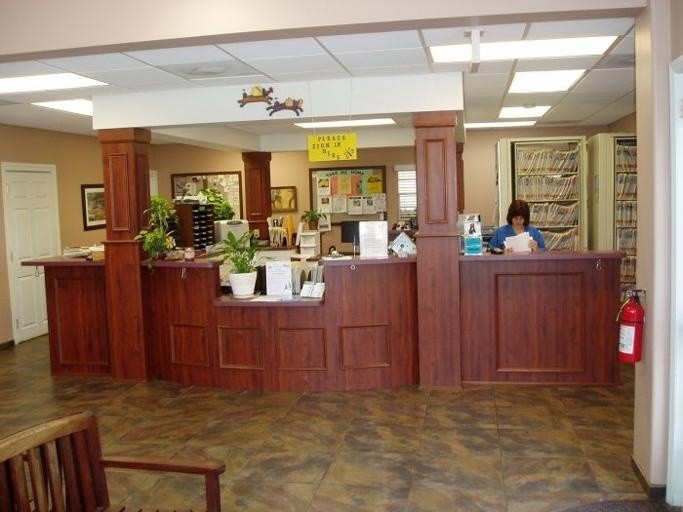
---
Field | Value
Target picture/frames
[269,186,298,213]
[308,165,388,226]
[80,183,106,232]
[169,170,243,221]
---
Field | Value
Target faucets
[352,234,356,259]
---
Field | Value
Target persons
[489,200,546,254]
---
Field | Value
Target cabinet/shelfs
[589,133,638,300]
[497,136,587,253]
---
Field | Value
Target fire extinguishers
[616,288,645,364]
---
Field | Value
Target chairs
[0,409,226,512]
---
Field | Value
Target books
[529,203,578,227]
[615,201,637,228]
[515,174,578,201]
[620,256,636,282]
[517,147,580,174]
[616,145,637,173]
[617,228,636,255]
[543,228,578,253]
[615,174,638,200]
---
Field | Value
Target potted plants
[299,209,327,231]
[218,230,260,300]
